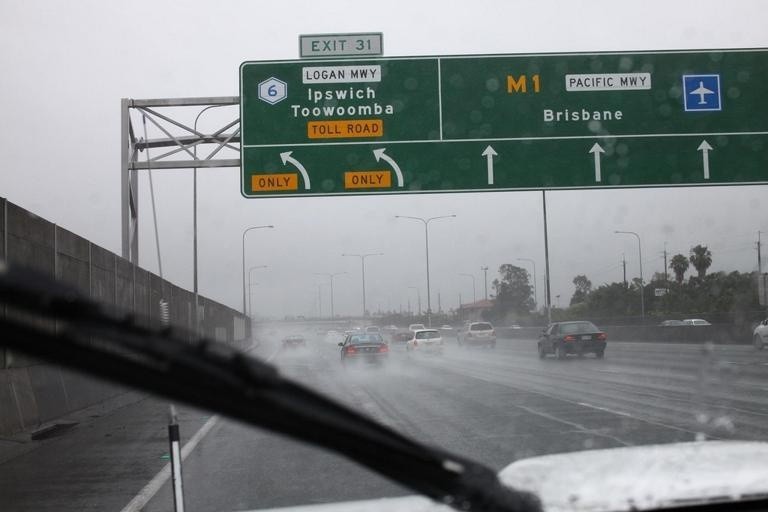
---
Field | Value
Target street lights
[314,214,535,316]
[614,231,645,324]
[243,225,274,317]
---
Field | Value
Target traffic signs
[239,32,767,202]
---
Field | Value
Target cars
[458,322,497,349]
[537,320,608,363]
[753,320,767,350]
[282,324,453,375]
[660,318,711,328]
